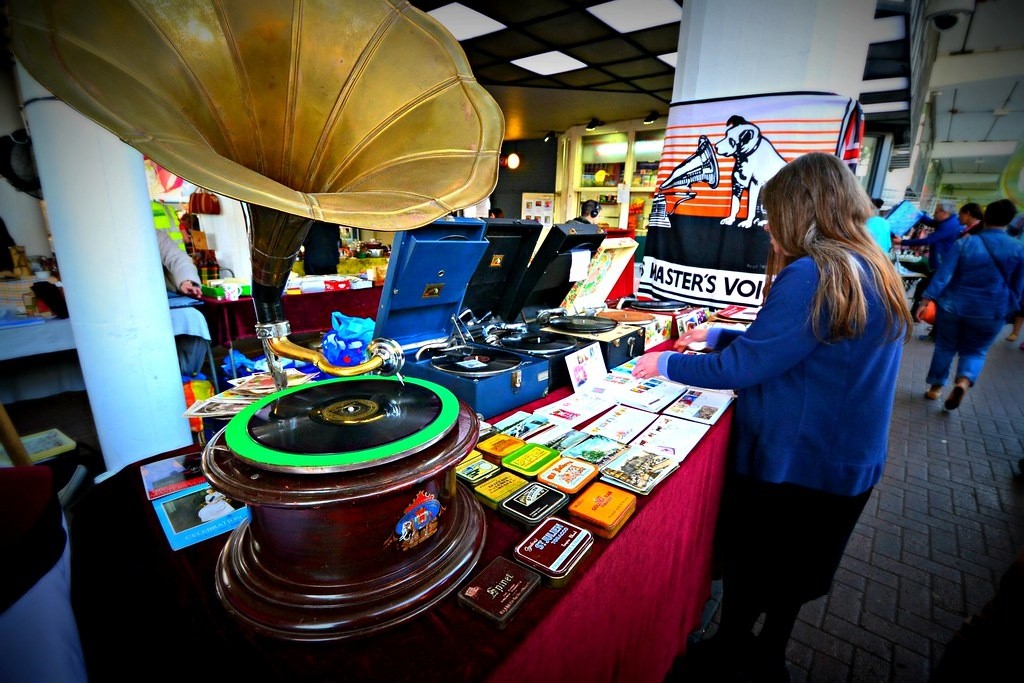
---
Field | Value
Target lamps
[644,110,669,125]
[585,116,606,131]
[544,131,565,143]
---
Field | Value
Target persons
[490,208,504,218]
[566,200,602,225]
[630,152,914,683]
[955,202,984,240]
[862,198,892,255]
[916,199,1024,410]
[303,220,341,275]
[891,199,962,342]
[155,228,211,376]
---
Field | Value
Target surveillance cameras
[924,0,975,31]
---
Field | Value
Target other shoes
[925,387,940,400]
[919,333,935,343]
[945,383,966,410]
[1006,333,1018,342]
[682,634,794,683]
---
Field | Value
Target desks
[178,274,384,356]
[62,296,736,683]
[0,293,221,468]
[291,257,389,280]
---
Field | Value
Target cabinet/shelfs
[552,117,670,237]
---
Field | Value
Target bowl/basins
[367,248,384,257]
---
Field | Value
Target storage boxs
[456,427,639,630]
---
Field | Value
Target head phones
[591,202,600,218]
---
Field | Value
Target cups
[222,283,243,301]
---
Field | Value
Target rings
[641,372,645,377]
